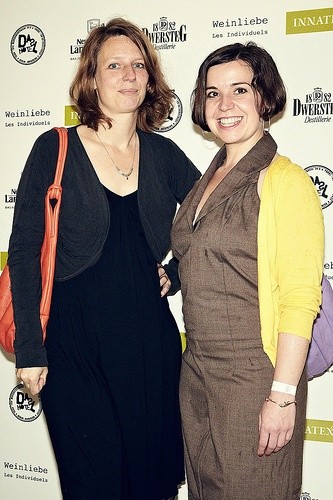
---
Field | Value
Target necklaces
[95,128,136,180]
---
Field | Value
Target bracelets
[264,397,298,408]
[270,380,298,395]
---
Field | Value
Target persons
[8,19,203,500]
[168,43,325,500]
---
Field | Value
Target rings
[162,276,169,280]
[20,380,24,385]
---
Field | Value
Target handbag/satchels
[0,126,68,355]
[306,276,333,379]
